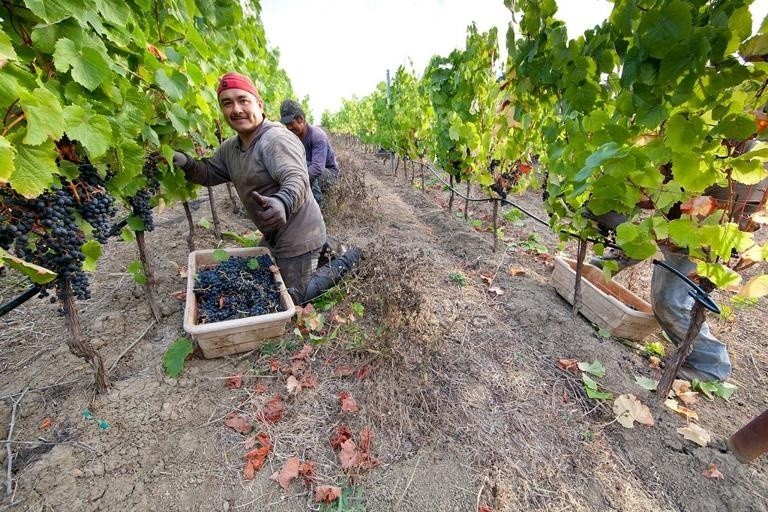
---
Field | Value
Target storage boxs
[183,247,296,359]
[549,255,658,342]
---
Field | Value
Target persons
[586,131,768,387]
[280,100,340,203]
[167,71,367,306]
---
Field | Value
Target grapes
[0,148,166,318]
[192,253,284,325]
[447,143,619,248]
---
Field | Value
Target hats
[280,100,300,124]
[218,72,258,97]
